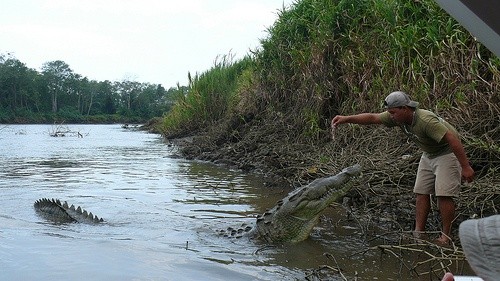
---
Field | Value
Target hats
[458,214,500,281]
[383,91,419,109]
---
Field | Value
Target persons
[441,215,500,281]
[331,91,475,241]
